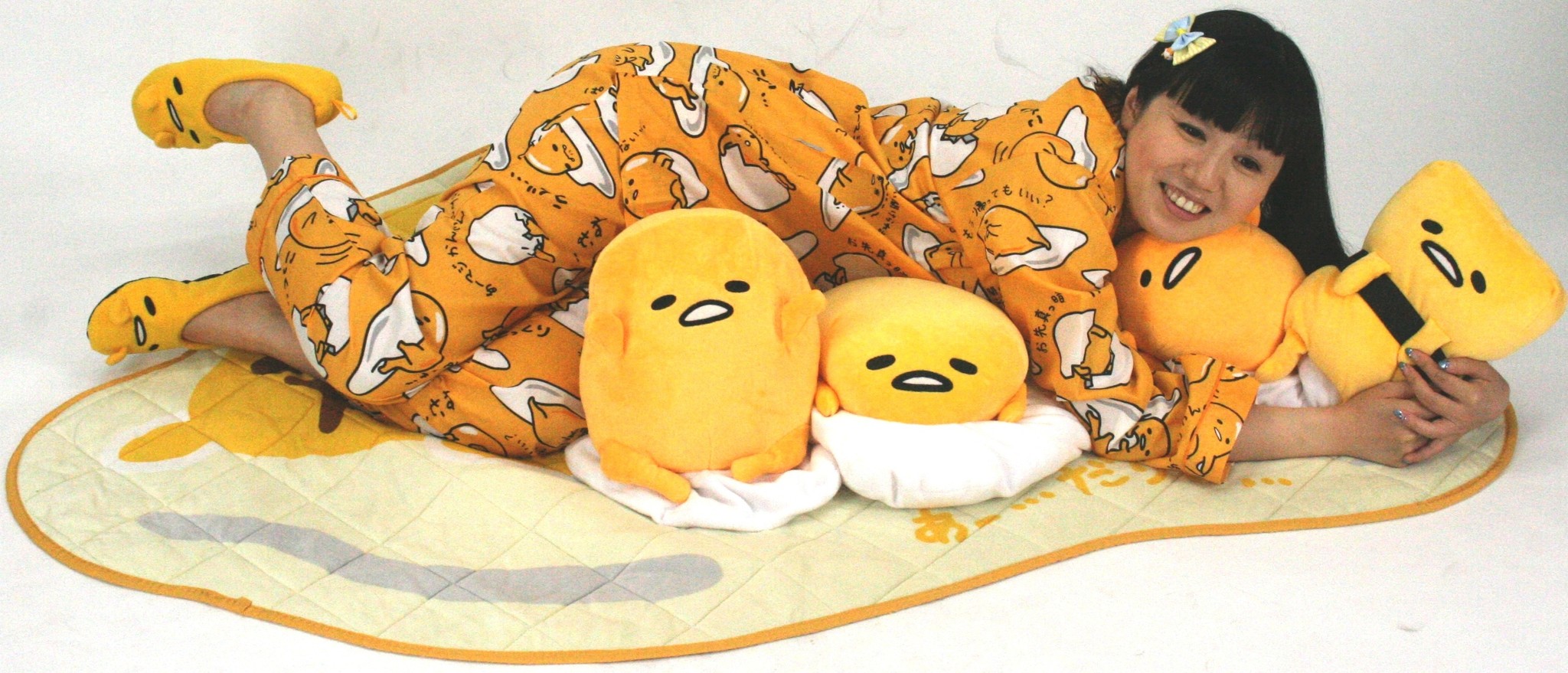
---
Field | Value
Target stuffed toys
[1254,160,1568,403]
[579,207,829,503]
[1110,206,1305,371]
[814,277,1030,423]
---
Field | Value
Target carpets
[6,144,1517,666]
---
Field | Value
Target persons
[86,9,1510,468]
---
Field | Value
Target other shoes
[129,59,357,150]
[87,262,269,366]
